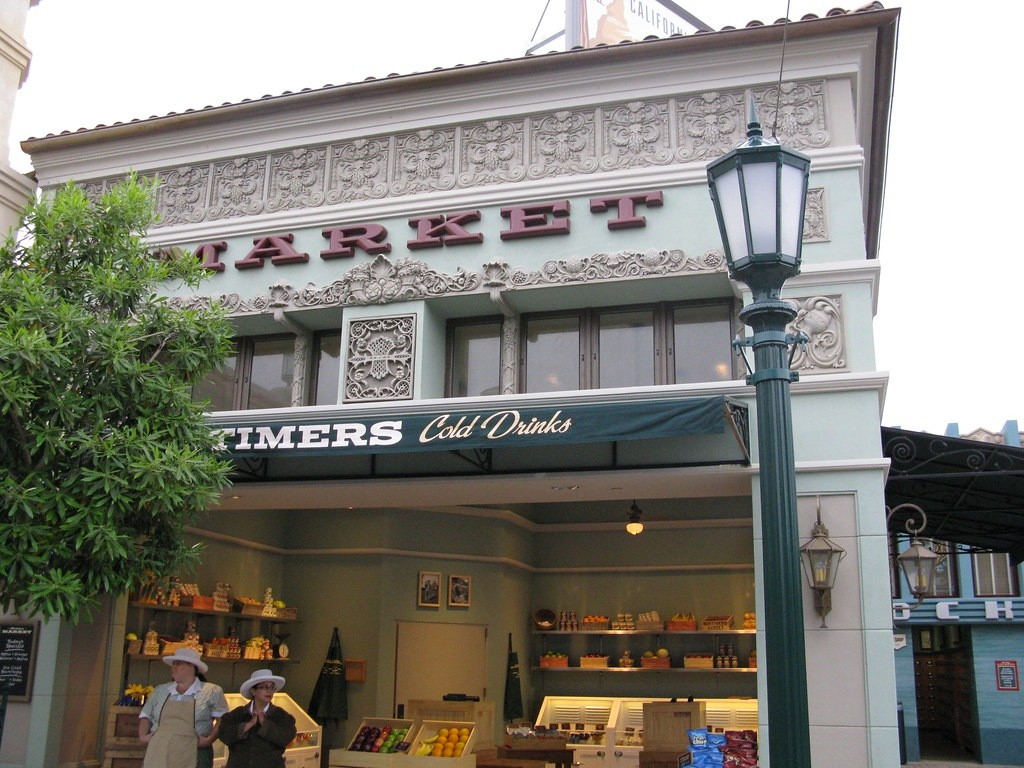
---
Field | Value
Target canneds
[716,643,737,668]
[224,626,241,659]
[182,620,203,657]
[259,638,273,660]
[611,612,635,630]
[559,610,578,631]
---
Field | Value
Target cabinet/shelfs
[528,629,766,673]
[106,601,324,768]
[544,745,644,768]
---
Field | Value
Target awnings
[209,396,749,482]
[881,427,1024,566]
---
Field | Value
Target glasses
[255,684,277,693]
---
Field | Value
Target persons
[218,669,296,768]
[138,649,230,768]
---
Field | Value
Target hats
[240,670,285,700]
[162,649,208,674]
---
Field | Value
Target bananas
[413,734,439,756]
[672,611,695,620]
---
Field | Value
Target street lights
[706,87,811,768]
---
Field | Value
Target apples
[349,724,408,753]
[642,649,668,659]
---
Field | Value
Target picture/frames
[448,574,471,607]
[0,618,42,702]
[418,571,443,607]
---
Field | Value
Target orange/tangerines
[431,728,469,758]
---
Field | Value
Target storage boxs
[539,656,569,668]
[580,656,611,668]
[640,654,671,668]
[329,718,566,768]
[579,615,611,630]
[667,620,699,631]
[701,615,734,630]
[683,652,714,669]
[634,620,665,631]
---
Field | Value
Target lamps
[800,495,847,628]
[625,500,644,535]
[886,503,938,610]
